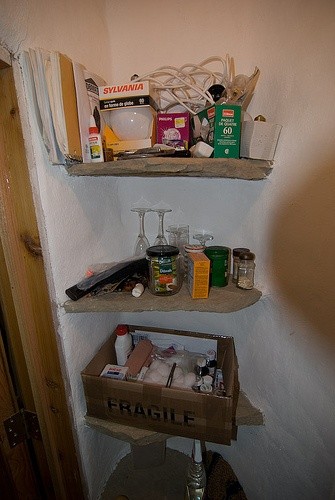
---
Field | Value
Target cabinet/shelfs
[58,156,279,447]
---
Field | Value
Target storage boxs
[187,252,211,300]
[238,119,284,162]
[156,113,191,150]
[97,80,163,161]
[99,364,130,380]
[125,339,153,378]
[189,103,243,160]
[103,148,114,163]
[78,322,240,446]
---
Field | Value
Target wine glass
[150,208,172,245]
[129,208,152,256]
[193,233,214,246]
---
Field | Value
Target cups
[167,223,190,280]
[184,244,205,280]
[204,246,231,288]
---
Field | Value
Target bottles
[115,324,133,366]
[231,247,250,283]
[235,251,256,289]
[192,349,227,397]
[87,115,104,163]
